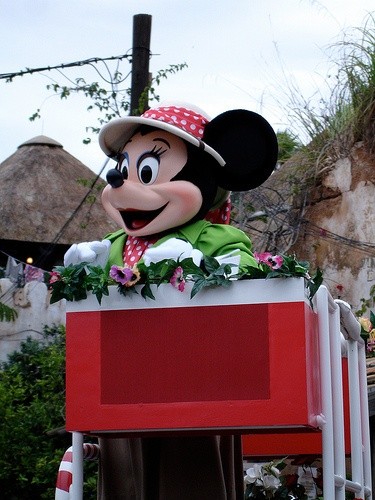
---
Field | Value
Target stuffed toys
[61,101,279,281]
